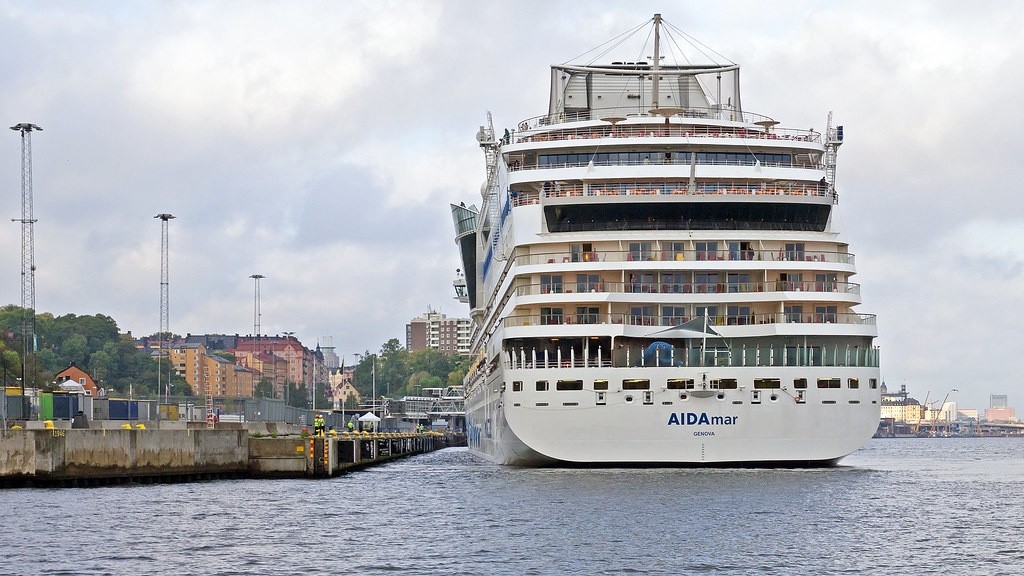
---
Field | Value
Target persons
[319,415,326,435]
[416,423,424,434]
[347,421,374,433]
[314,414,320,434]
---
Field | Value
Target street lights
[9,120,43,421]
[153,212,178,415]
[250,273,266,395]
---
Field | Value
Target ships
[445,12,882,468]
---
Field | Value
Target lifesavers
[563,360,571,368]
